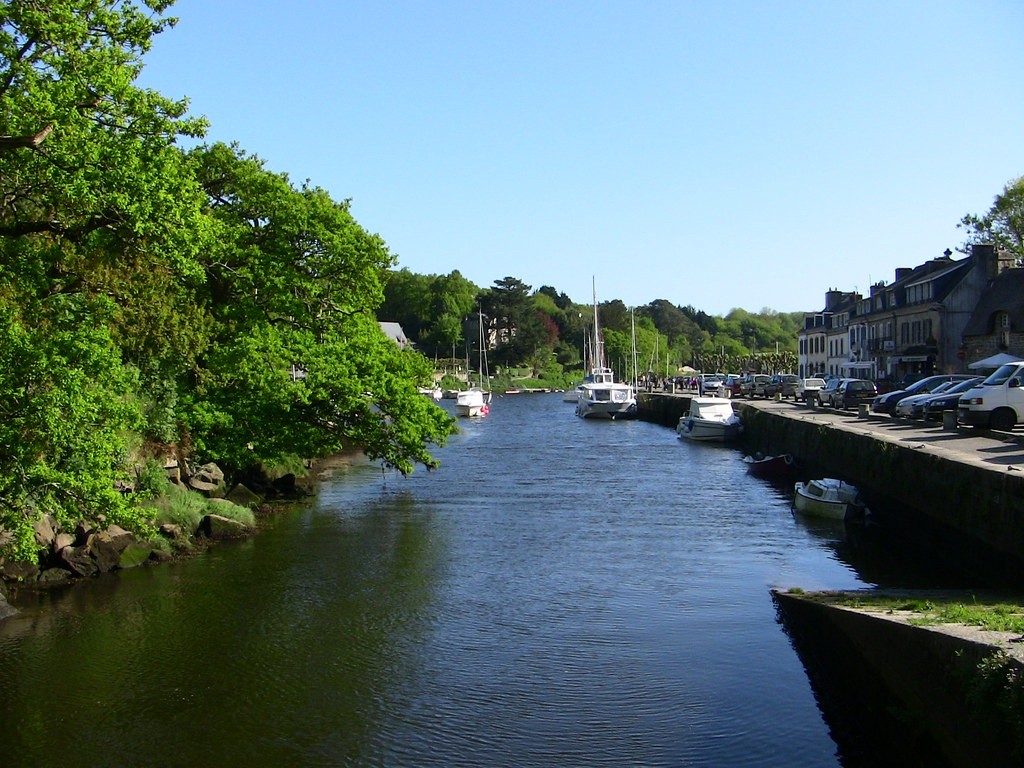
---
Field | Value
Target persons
[640,371,699,390]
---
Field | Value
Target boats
[455,391,486,417]
[793,477,866,524]
[741,450,794,474]
[676,398,743,444]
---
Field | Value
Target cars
[739,374,771,399]
[817,378,856,407]
[958,361,1024,432]
[874,373,1019,426]
[684,376,691,383]
[698,374,740,385]
[811,373,845,383]
[702,377,724,395]
[763,374,801,399]
[725,378,745,398]
[793,379,827,402]
[832,380,878,411]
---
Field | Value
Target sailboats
[574,274,639,420]
[562,328,589,404]
[467,303,492,404]
